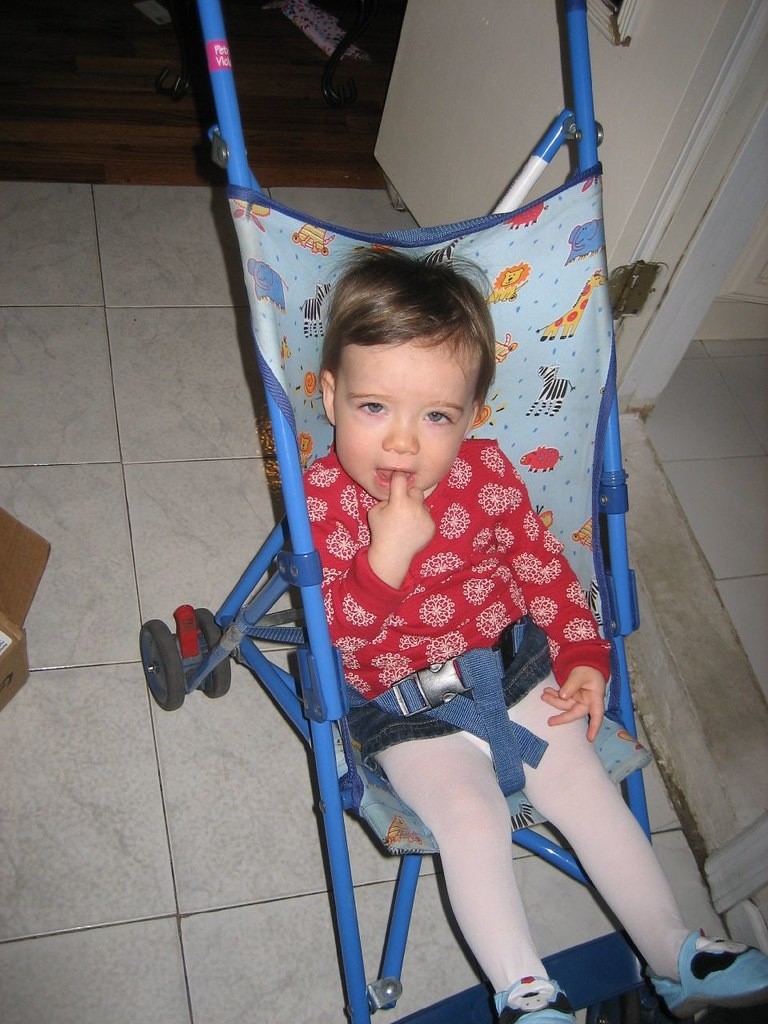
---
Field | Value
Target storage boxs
[1,506,51,705]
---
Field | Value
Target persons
[304,243,767,1024]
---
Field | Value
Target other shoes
[645,928,768,1015]
[493,975,577,1024]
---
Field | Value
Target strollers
[139,0,699,1024]
